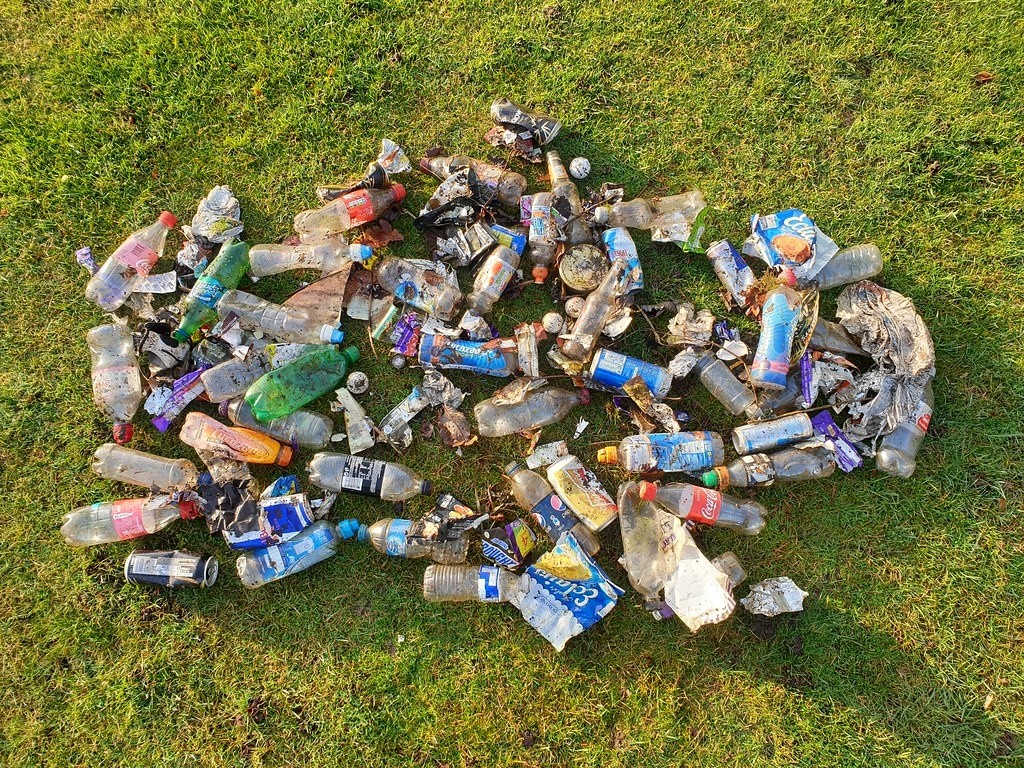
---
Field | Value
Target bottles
[358,518,470,565]
[217,290,345,344]
[200,343,306,403]
[243,346,361,420]
[294,184,406,244]
[546,150,593,245]
[418,334,524,377]
[466,245,520,318]
[420,154,528,206]
[61,495,180,546]
[171,237,251,343]
[218,396,332,449]
[248,244,372,277]
[474,385,591,437]
[308,452,433,501]
[504,461,600,556]
[86,211,178,311]
[178,411,293,466]
[85,324,142,444]
[91,444,212,494]
[530,192,936,621]
[424,565,530,603]
[363,254,465,322]
[235,518,360,589]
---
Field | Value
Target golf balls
[569,156,591,179]
[347,371,369,394]
[541,312,563,334]
[564,297,586,318]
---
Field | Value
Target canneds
[546,453,618,532]
[705,237,759,307]
[316,161,390,206]
[221,492,315,549]
[488,97,563,147]
[602,226,645,291]
[589,348,673,401]
[733,412,814,455]
[124,550,218,590]
